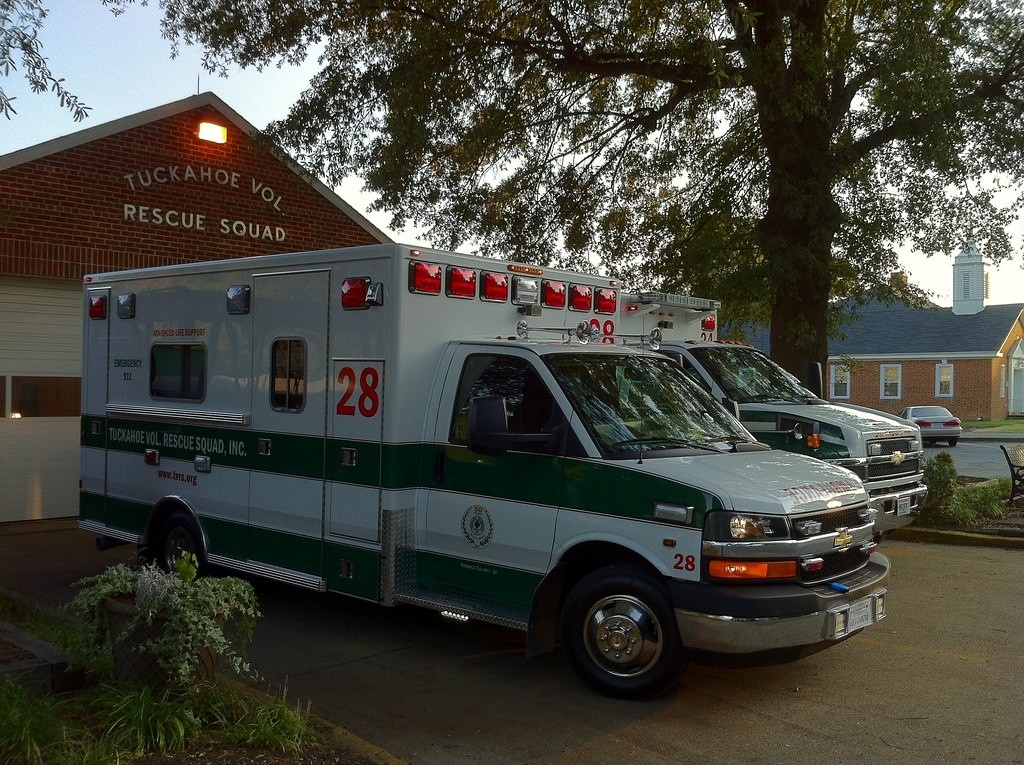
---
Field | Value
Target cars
[897,406,963,447]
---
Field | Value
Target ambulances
[77,243,894,700]
[616,290,928,547]
